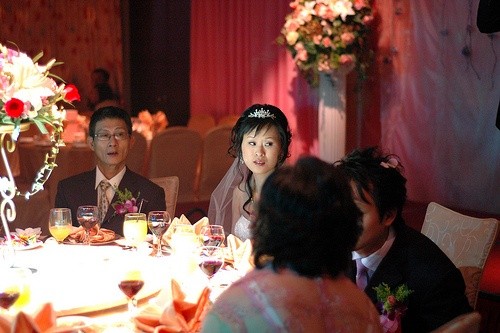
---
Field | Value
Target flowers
[0,41,81,200]
[374,281,414,333]
[277,0,375,89]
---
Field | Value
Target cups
[197,224,226,281]
[0,279,20,311]
[48,207,73,244]
[122,213,148,247]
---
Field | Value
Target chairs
[0,126,241,233]
[421,201,500,333]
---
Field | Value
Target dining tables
[0,236,255,333]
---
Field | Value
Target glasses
[94,132,131,141]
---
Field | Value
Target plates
[63,228,120,246]
[0,237,44,250]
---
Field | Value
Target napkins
[0,227,255,333]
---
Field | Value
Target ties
[355,258,368,291]
[97,181,110,227]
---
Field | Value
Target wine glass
[147,211,171,257]
[77,206,99,246]
[118,269,145,315]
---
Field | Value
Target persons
[85,68,110,111]
[206,103,292,242]
[54,105,166,237]
[89,83,118,110]
[333,147,473,333]
[200,154,382,333]
